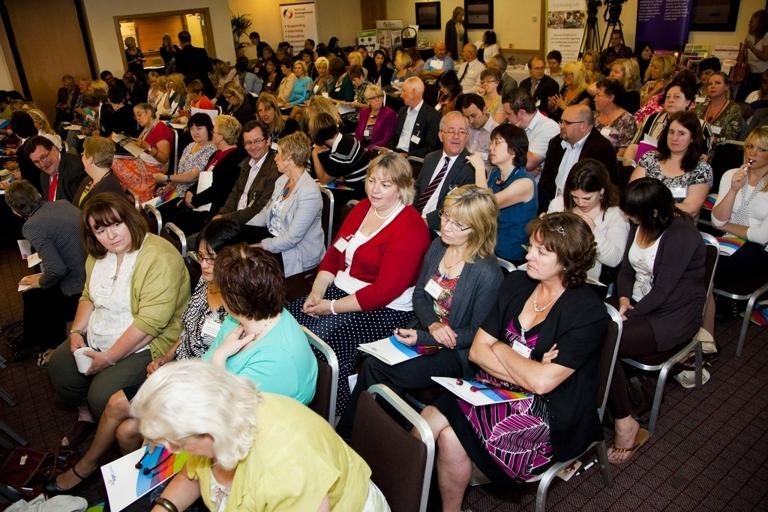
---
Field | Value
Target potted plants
[231,12,253,58]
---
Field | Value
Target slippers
[607,427,649,464]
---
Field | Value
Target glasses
[558,120,584,126]
[243,137,266,145]
[225,95,233,100]
[32,150,51,165]
[439,210,472,231]
[196,253,215,265]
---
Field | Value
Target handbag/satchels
[728,41,750,85]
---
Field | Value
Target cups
[73,347,94,374]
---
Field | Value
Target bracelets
[151,495,180,512]
[331,298,338,316]
[154,151,159,157]
[99,350,117,366]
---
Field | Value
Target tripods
[601,20,625,53]
[577,17,600,61]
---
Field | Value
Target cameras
[603,0,627,24]
[586,0,602,17]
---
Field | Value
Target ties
[416,157,451,215]
[530,81,540,97]
[459,64,469,84]
[48,175,57,202]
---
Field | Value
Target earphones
[135,447,174,475]
[456,377,496,392]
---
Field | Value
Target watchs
[147,146,153,153]
[67,328,85,337]
[167,175,172,183]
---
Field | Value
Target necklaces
[532,281,567,312]
[373,200,403,220]
[443,245,475,270]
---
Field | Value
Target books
[337,101,355,115]
[100,443,199,512]
[112,131,146,158]
[710,233,745,258]
[192,170,213,212]
[141,190,179,210]
[192,171,214,212]
[191,106,219,129]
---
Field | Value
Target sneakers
[690,342,716,356]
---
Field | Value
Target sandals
[45,462,99,495]
[60,421,101,448]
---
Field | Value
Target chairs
[301,325,339,428]
[168,128,178,176]
[320,187,334,250]
[183,251,202,295]
[160,223,187,257]
[620,233,720,438]
[61,140,69,153]
[125,187,140,210]
[140,203,162,235]
[352,384,435,512]
[524,302,623,512]
[405,257,517,409]
[710,139,744,195]
[712,283,767,357]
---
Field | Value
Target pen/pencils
[744,160,753,173]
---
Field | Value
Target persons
[455,42,486,96]
[280,60,314,117]
[53,75,79,134]
[311,112,375,239]
[601,177,708,465]
[436,70,465,116]
[385,76,442,170]
[640,54,678,106]
[249,31,276,65]
[204,56,280,101]
[290,57,334,123]
[519,56,560,121]
[445,7,470,62]
[550,59,595,123]
[337,66,379,134]
[355,85,398,159]
[235,44,247,61]
[538,104,616,215]
[244,130,327,281]
[518,56,560,121]
[67,80,110,136]
[135,241,319,454]
[22,135,84,203]
[111,103,174,203]
[691,72,743,146]
[626,110,714,229]
[549,62,592,124]
[4,178,93,363]
[159,34,181,73]
[154,114,243,235]
[486,53,518,104]
[410,211,609,512]
[423,42,454,78]
[124,37,147,77]
[619,80,712,190]
[601,30,634,76]
[128,358,393,512]
[264,37,348,67]
[543,50,564,93]
[455,93,499,176]
[43,216,251,496]
[100,70,146,106]
[413,111,490,239]
[257,93,300,144]
[591,78,637,158]
[47,191,194,451]
[356,46,425,85]
[336,184,505,444]
[501,88,561,186]
[76,93,117,151]
[694,56,734,102]
[638,42,655,81]
[151,112,218,198]
[734,9,767,102]
[146,71,188,121]
[189,120,280,260]
[170,79,215,159]
[284,151,432,417]
[701,124,767,354]
[175,30,211,98]
[607,58,640,116]
[475,68,508,124]
[0,90,55,134]
[465,124,540,266]
[92,87,138,155]
[546,157,634,307]
[582,50,606,83]
[221,80,257,131]
[0,110,45,197]
[323,56,353,108]
[275,60,298,105]
[71,135,122,212]
[474,29,499,66]
